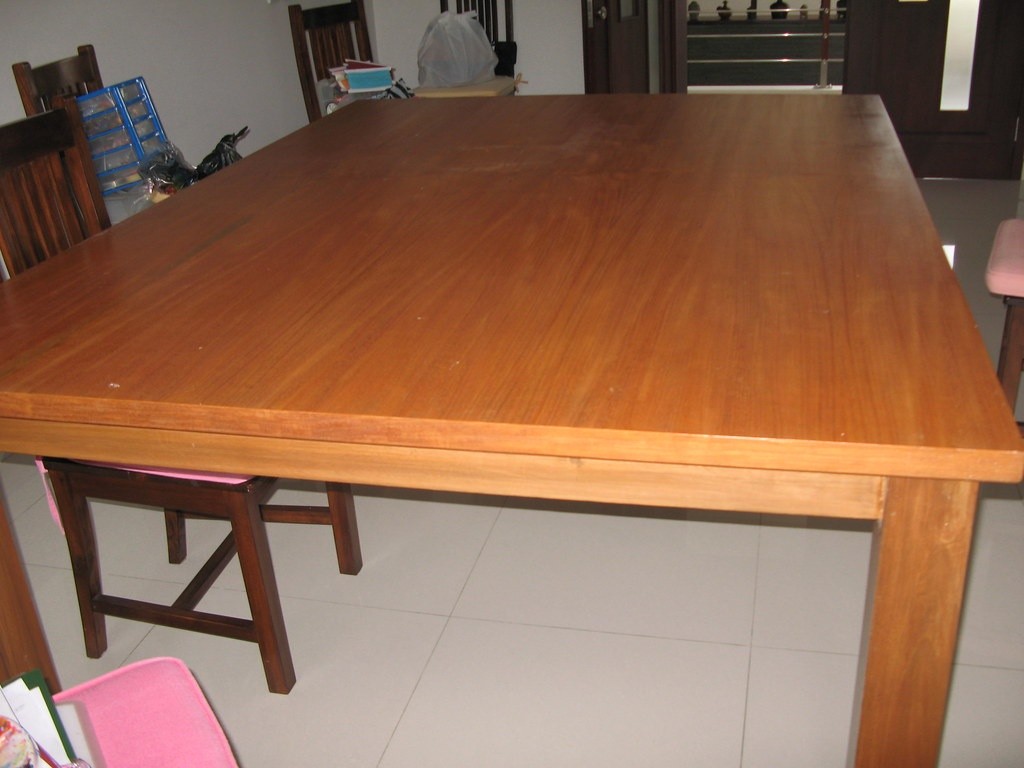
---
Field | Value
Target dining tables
[1,92,1022,767]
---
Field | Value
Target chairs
[11,45,106,117]
[409,1,518,98]
[0,96,365,697]
[287,1,376,125]
[983,218,1024,421]
